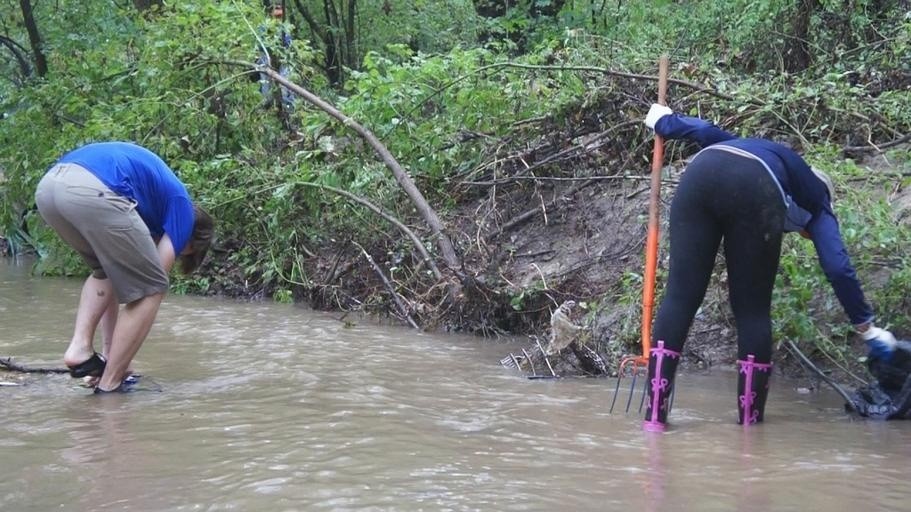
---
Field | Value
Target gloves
[859,322,897,354]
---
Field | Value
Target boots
[643,340,681,433]
[735,355,774,426]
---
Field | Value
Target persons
[250,3,298,116]
[35,139,215,396]
[636,99,903,436]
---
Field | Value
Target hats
[812,169,836,214]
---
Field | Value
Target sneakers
[93,382,147,394]
[68,351,106,377]
